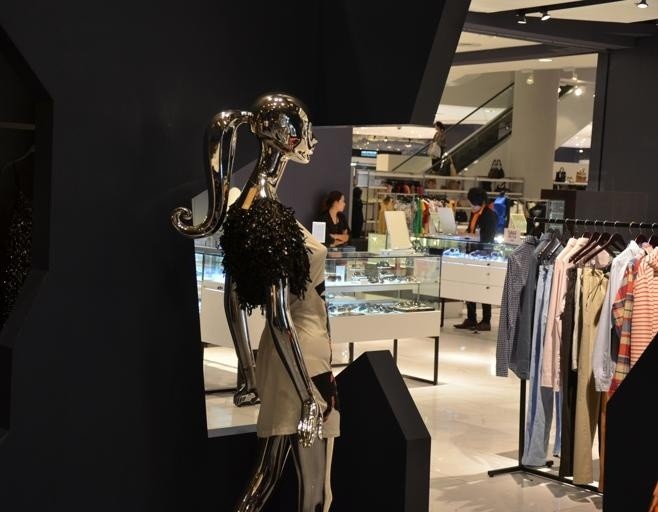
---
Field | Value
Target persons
[326,247,347,281]
[169,91,341,511]
[352,187,364,239]
[453,187,499,332]
[320,190,351,249]
[492,191,509,234]
[431,120,446,172]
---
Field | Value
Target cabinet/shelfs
[357,169,525,231]
[321,247,442,387]
[385,232,521,331]
[195,244,268,395]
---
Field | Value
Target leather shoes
[453,319,491,330]
